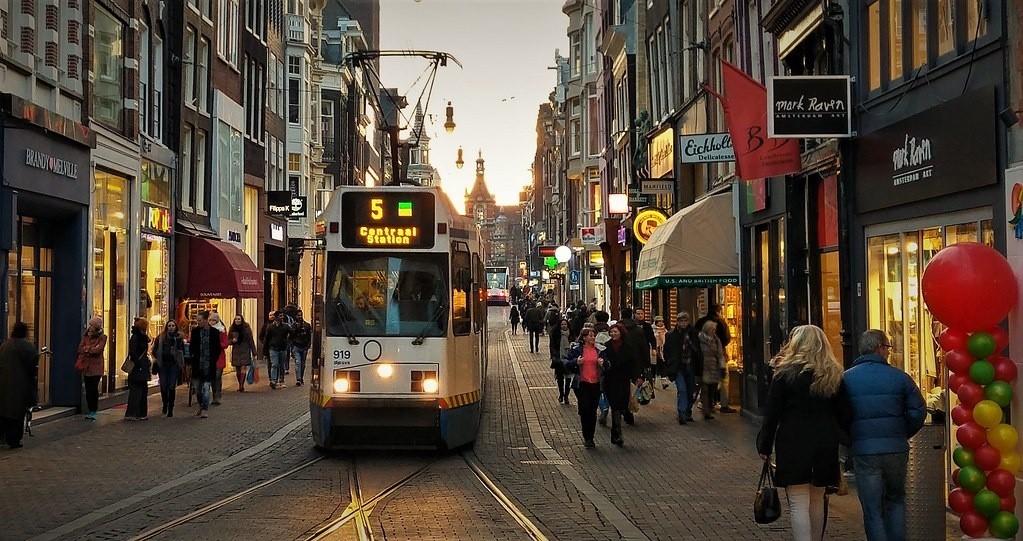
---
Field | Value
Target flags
[718,57,803,181]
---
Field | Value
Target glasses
[882,344,892,351]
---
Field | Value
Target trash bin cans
[901,421,948,541]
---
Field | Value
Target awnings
[635,192,740,289]
[174,231,265,300]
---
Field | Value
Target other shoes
[279,382,283,388]
[720,406,738,413]
[559,393,564,402]
[11,442,23,449]
[600,410,608,427]
[564,396,569,404]
[685,410,694,422]
[616,439,624,447]
[678,416,687,425]
[237,386,244,392]
[584,439,596,447]
[86,407,210,421]
[705,415,715,421]
[211,401,221,406]
[296,381,302,386]
[270,382,276,389]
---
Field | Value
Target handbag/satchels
[628,382,639,413]
[121,358,134,373]
[638,378,653,405]
[76,358,86,370]
[754,457,781,524]
[247,359,259,384]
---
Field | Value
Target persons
[228,313,258,392]
[77,317,107,419]
[152,319,186,420]
[599,324,635,445]
[280,302,297,324]
[401,272,437,301]
[509,284,668,427]
[189,311,229,417]
[562,327,611,449]
[663,302,737,425]
[123,316,151,421]
[259,310,312,391]
[842,329,928,541]
[756,324,845,541]
[0,321,40,449]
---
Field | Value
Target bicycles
[21,346,56,441]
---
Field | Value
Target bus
[285,47,491,458]
[486,266,511,306]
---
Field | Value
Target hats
[134,317,149,329]
[654,316,664,321]
[88,316,103,329]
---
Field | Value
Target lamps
[444,102,456,133]
[456,146,464,169]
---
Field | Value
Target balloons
[921,241,1018,332]
[938,325,1023,541]
